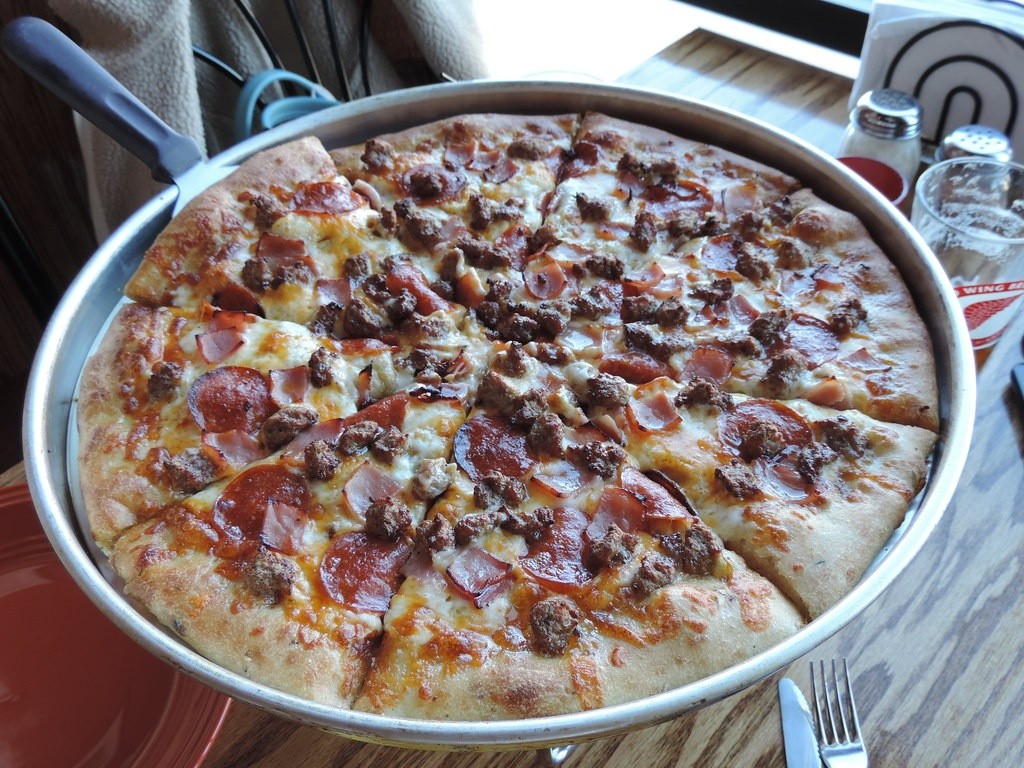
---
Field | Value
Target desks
[0,27,1024,768]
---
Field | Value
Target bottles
[929,123,1013,210]
[838,88,924,192]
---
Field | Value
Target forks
[810,657,868,768]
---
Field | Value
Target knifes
[778,678,825,768]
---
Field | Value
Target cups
[910,156,1024,373]
[836,156,909,210]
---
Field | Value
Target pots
[1,15,979,751]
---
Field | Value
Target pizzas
[74,113,938,723]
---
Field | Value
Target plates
[0,482,232,767]
